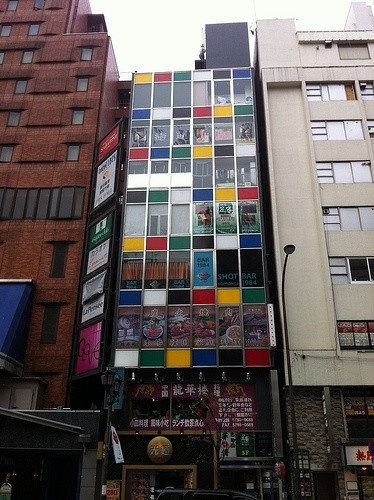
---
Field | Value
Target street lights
[281,244,303,500]
[100,367,123,500]
